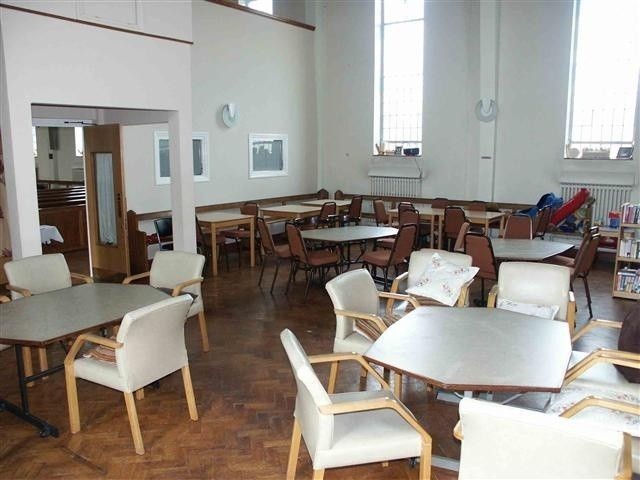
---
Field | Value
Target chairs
[218,203,263,268]
[64,295,199,455]
[541,225,601,317]
[383,248,474,393]
[453,397,633,480]
[397,202,415,223]
[317,189,329,200]
[373,199,389,227]
[256,217,296,295]
[0,295,35,387]
[487,262,577,340]
[545,308,640,438]
[443,207,466,250]
[362,223,418,292]
[431,198,449,224]
[3,253,95,380]
[325,267,421,401]
[279,328,432,480]
[468,201,488,211]
[122,251,210,352]
[284,222,340,304]
[503,214,533,240]
[334,190,344,200]
[195,216,230,274]
[154,216,203,255]
[463,233,498,300]
[348,196,362,227]
[317,202,336,229]
[376,209,419,251]
[532,206,552,240]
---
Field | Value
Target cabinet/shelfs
[612,224,640,300]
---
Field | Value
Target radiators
[558,169,638,227]
[368,168,424,198]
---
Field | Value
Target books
[616,266,640,294]
[623,202,640,224]
[619,239,640,259]
